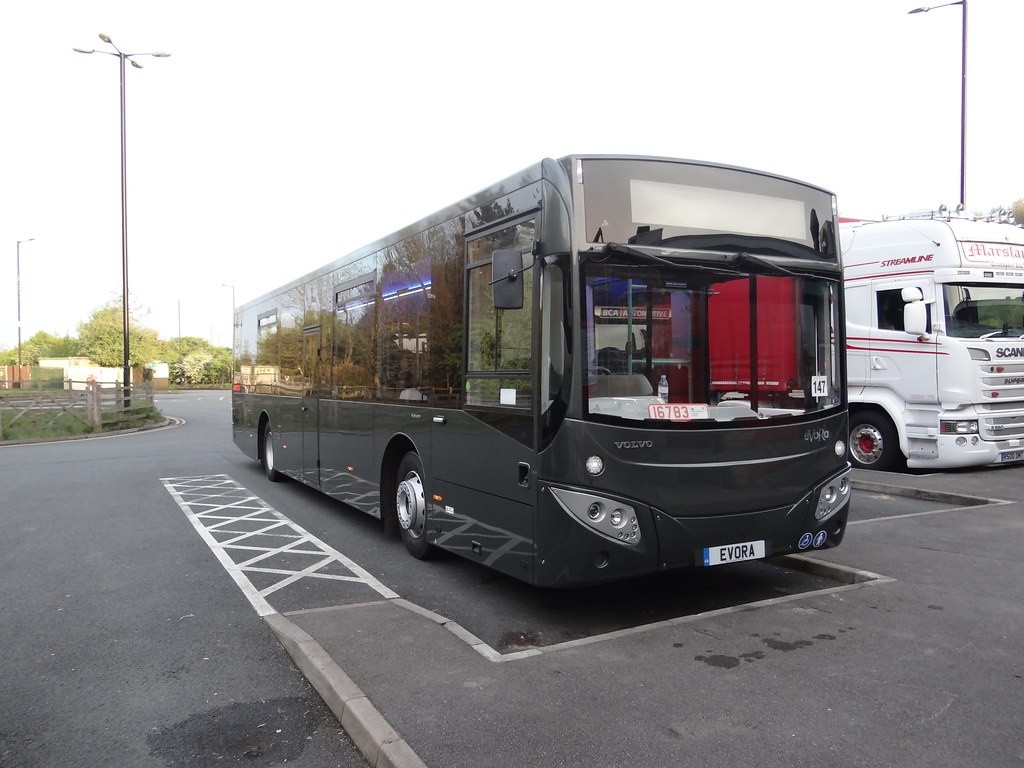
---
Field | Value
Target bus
[231,155,851,589]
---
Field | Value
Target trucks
[650,214,1024,469]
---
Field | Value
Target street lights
[222,282,235,311]
[17,238,34,379]
[908,0,967,210]
[73,33,172,414]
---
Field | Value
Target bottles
[657,375,668,404]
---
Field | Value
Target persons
[375,348,426,390]
